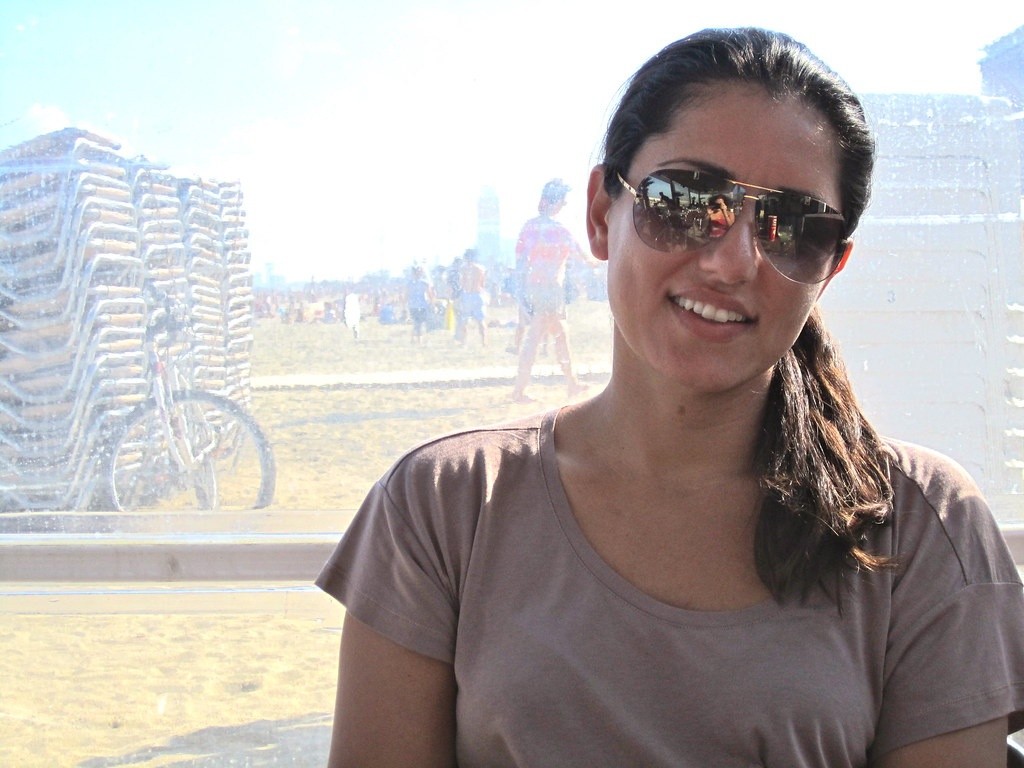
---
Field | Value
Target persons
[647,191,734,240]
[254,181,574,355]
[314,24,1024,768]
[507,176,599,402]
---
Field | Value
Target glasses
[616,169,850,285]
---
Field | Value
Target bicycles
[96,268,278,512]
